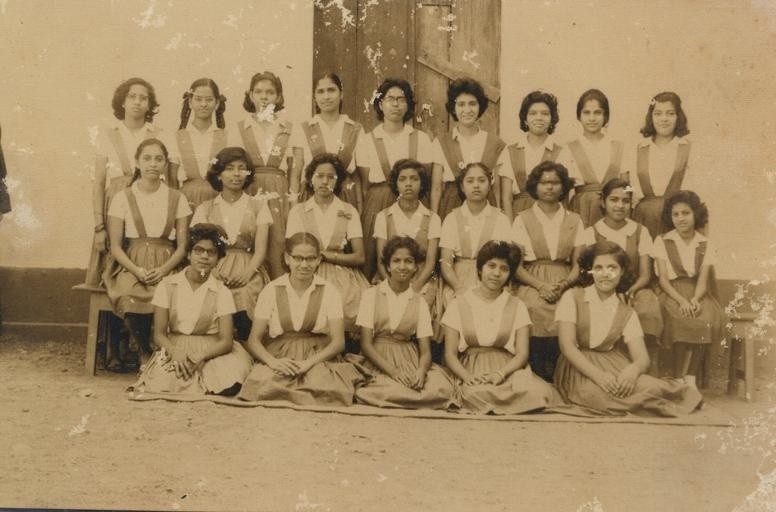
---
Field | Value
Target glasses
[192,245,218,257]
[381,95,408,104]
[288,252,317,263]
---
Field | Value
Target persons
[126,223,255,394]
[86,76,725,387]
[441,239,565,416]
[554,241,704,419]
[235,231,376,408]
[354,234,452,409]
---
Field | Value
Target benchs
[71,279,758,403]
